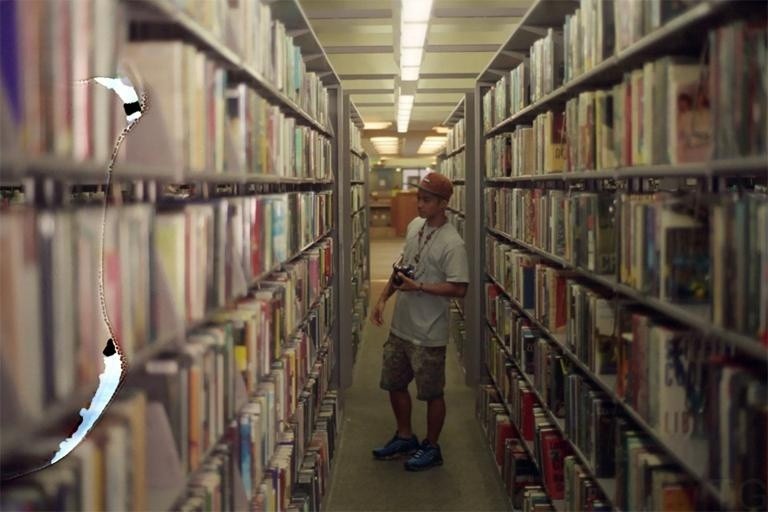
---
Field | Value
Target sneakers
[404,438,443,472]
[372,433,420,461]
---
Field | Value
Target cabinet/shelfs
[2,1,370,512]
[438,0,766,511]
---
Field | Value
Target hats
[408,172,453,201]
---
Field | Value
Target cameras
[393,263,414,285]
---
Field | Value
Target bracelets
[417,283,423,291]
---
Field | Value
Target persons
[367,171,471,470]
